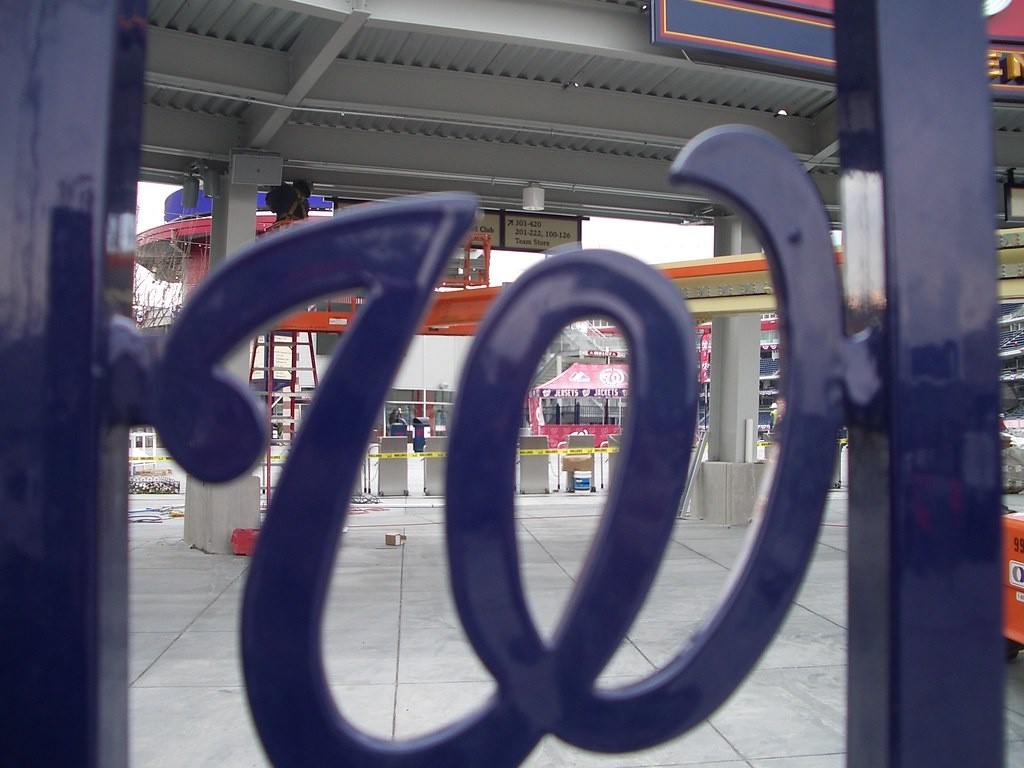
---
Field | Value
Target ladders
[249,330,319,514]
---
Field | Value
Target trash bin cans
[413,417,431,452]
[840,444,849,488]
[390,418,407,436]
[762,433,774,460]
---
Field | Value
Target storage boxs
[385,532,407,546]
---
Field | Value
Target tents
[536,363,629,425]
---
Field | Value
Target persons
[769,403,777,429]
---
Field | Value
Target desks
[540,424,620,449]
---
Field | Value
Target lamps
[202,159,221,199]
[180,171,200,209]
[522,181,545,211]
